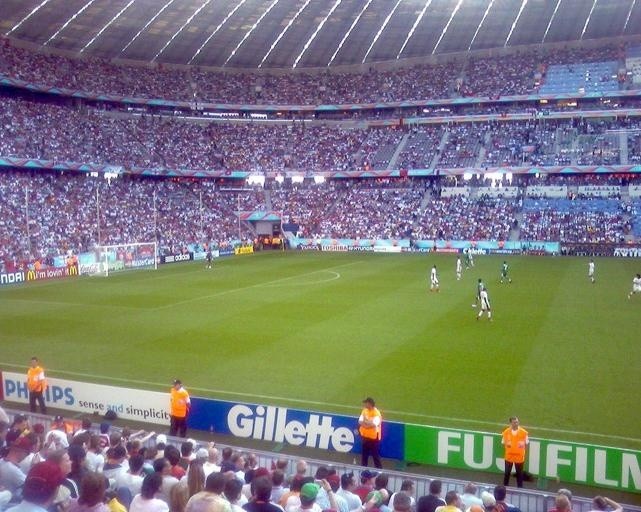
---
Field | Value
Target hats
[24,460,65,495]
[362,398,375,403]
[299,482,321,501]
[362,470,378,479]
[172,380,181,384]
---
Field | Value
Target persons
[500,260,512,284]
[1,408,522,512]
[587,259,596,283]
[455,255,463,281]
[430,264,441,293]
[547,488,578,512]
[587,495,624,512]
[628,273,641,300]
[501,415,530,488]
[169,378,192,437]
[471,278,485,309]
[25,356,48,415]
[2,37,640,269]
[476,287,493,322]
[357,397,383,469]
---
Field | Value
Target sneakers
[592,280,595,283]
[500,280,504,283]
[627,295,630,299]
[431,289,434,291]
[488,318,494,321]
[509,279,512,282]
[436,288,439,291]
[476,316,482,322]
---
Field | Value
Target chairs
[472,183,641,247]
[360,116,639,171]
[533,46,640,99]
